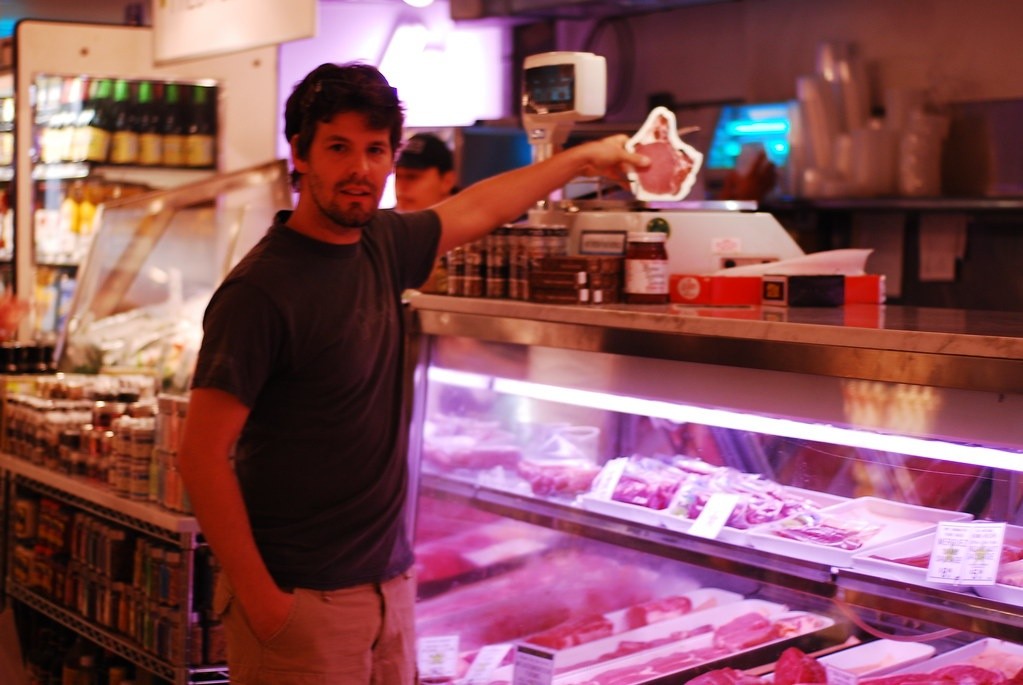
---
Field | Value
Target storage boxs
[761,274,886,305]
[531,253,621,305]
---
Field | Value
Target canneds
[440,220,569,301]
[54,400,161,502]
[623,231,669,304]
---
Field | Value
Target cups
[781,39,952,199]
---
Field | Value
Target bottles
[24,72,216,172]
[60,514,228,669]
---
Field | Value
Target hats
[396,133,453,171]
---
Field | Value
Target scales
[520,50,809,275]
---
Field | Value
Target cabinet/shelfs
[398,294,1023,685]
[0,19,280,338]
[0,450,231,685]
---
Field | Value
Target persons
[177,62,651,685]
[395,132,458,214]
